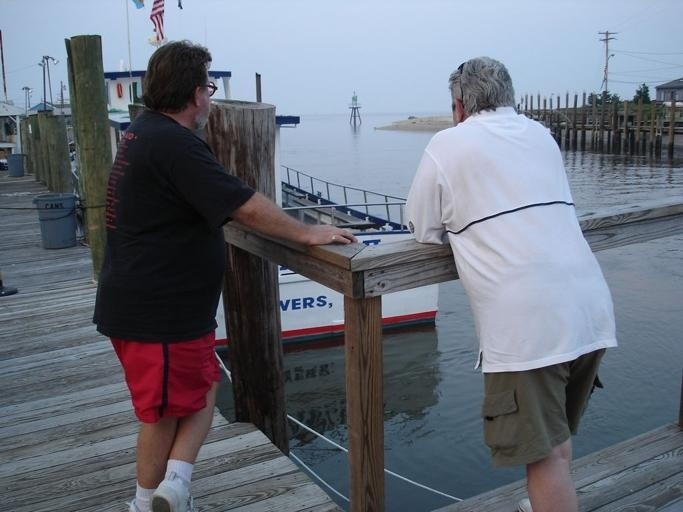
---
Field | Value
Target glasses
[191,81,218,98]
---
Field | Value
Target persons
[403,54,621,512]
[90,38,358,512]
[0,279,18,298]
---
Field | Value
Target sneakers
[128,478,197,512]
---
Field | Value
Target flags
[131,0,146,10]
[149,1,164,44]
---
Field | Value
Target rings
[332,234,338,243]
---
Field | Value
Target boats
[103,69,442,352]
[0,29,76,175]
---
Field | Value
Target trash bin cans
[32,193,80,249]
[4,154,26,177]
